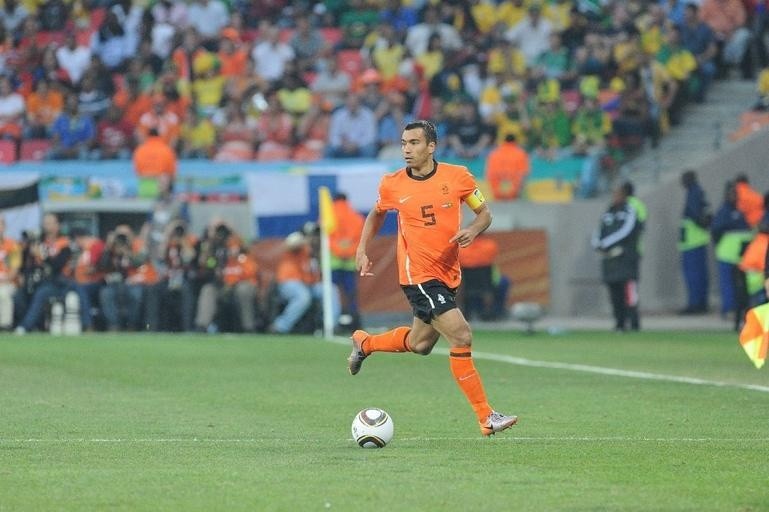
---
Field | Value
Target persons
[1,0,769,332]
[345,120,520,437]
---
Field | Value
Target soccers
[351,406,395,448]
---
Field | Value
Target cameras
[175,225,183,236]
[216,224,227,237]
[116,235,126,246]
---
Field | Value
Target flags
[737,301,769,372]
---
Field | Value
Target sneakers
[345,329,371,375]
[479,408,520,437]
